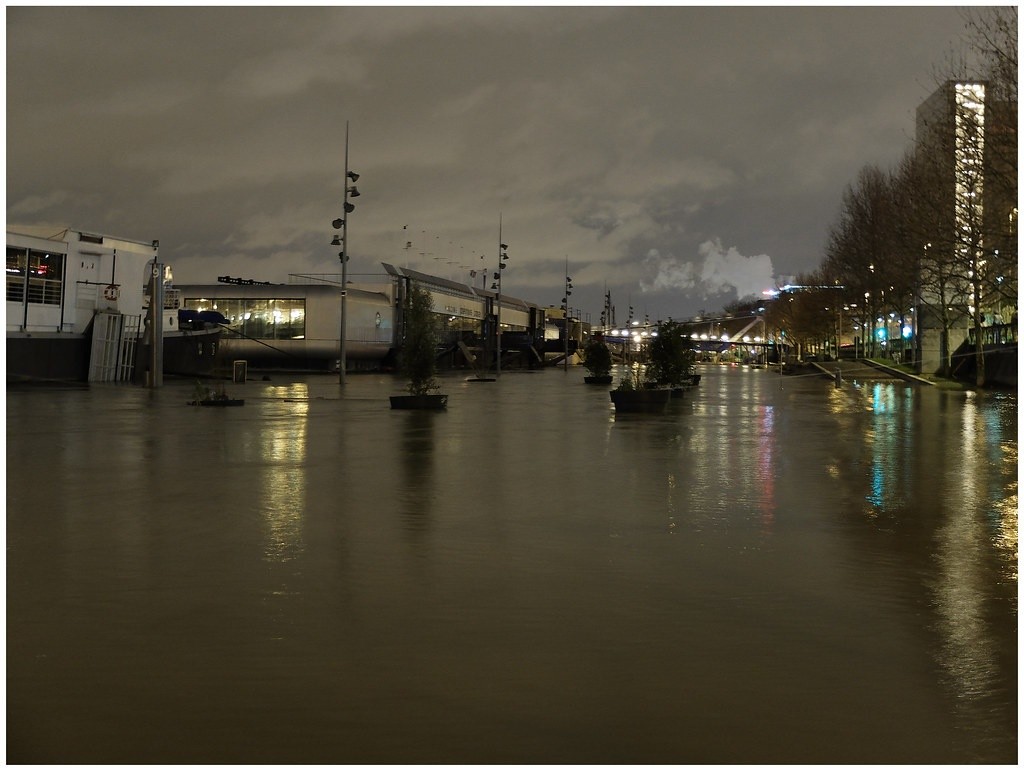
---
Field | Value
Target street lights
[1008,208,1018,279]
[625,304,633,367]
[600,280,611,344]
[494,212,509,377]
[559,255,573,372]
[330,120,360,385]
[910,243,932,367]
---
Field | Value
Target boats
[7,225,230,384]
